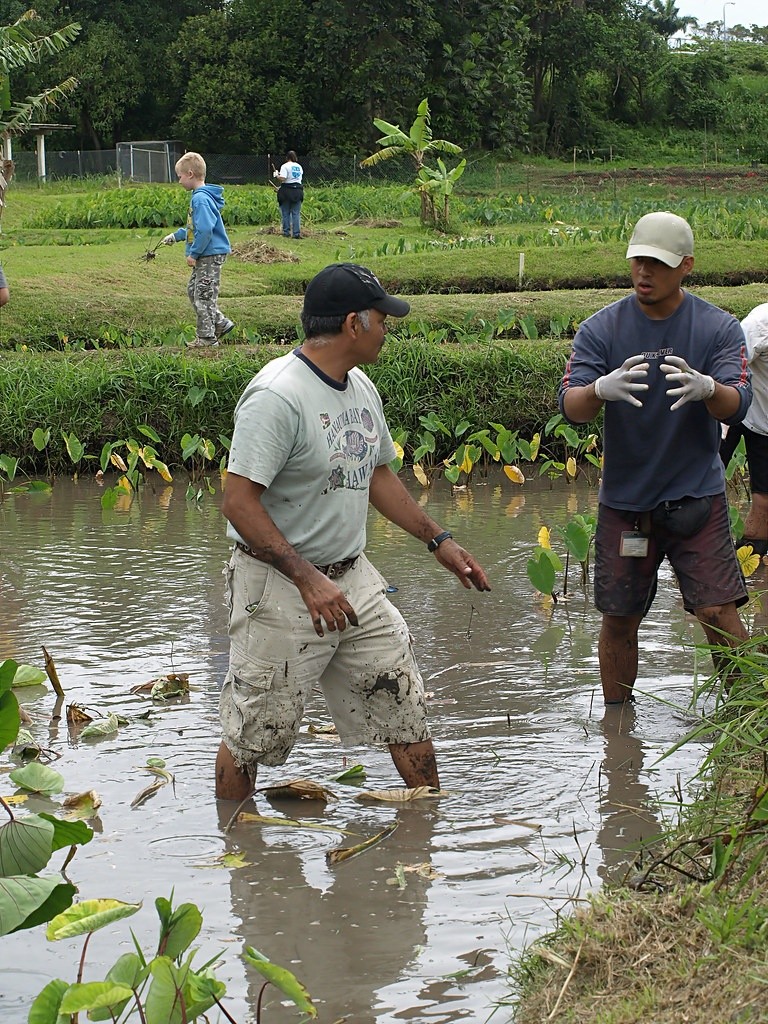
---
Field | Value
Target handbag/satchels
[658,496,713,540]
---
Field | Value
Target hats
[303,262,410,318]
[626,210,693,269]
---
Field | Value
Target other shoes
[184,335,219,349]
[293,235,303,239]
[283,234,291,238]
[213,317,236,340]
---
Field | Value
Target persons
[162,151,234,349]
[737,304,768,564]
[273,150,303,239]
[216,263,491,802]
[559,212,753,703]
[0,267,9,306]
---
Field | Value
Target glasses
[245,601,261,614]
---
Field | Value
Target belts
[236,539,360,578]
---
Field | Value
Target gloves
[273,170,278,177]
[594,354,650,408]
[160,232,176,246]
[659,355,716,411]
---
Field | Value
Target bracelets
[428,530,453,553]
